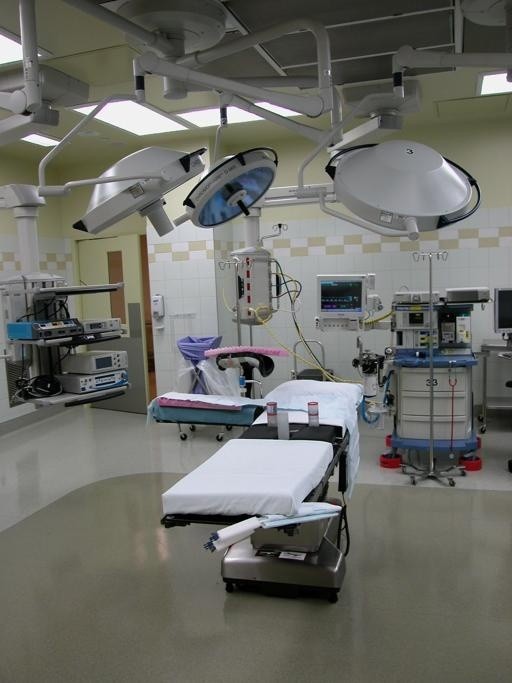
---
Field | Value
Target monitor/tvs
[316,274,367,318]
[494,288,512,334]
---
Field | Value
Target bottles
[308,402,320,427]
[266,401,277,427]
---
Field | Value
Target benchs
[147,395,263,442]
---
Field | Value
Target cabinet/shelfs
[380,348,483,471]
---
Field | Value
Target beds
[161,380,365,604]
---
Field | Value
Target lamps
[2,2,480,238]
